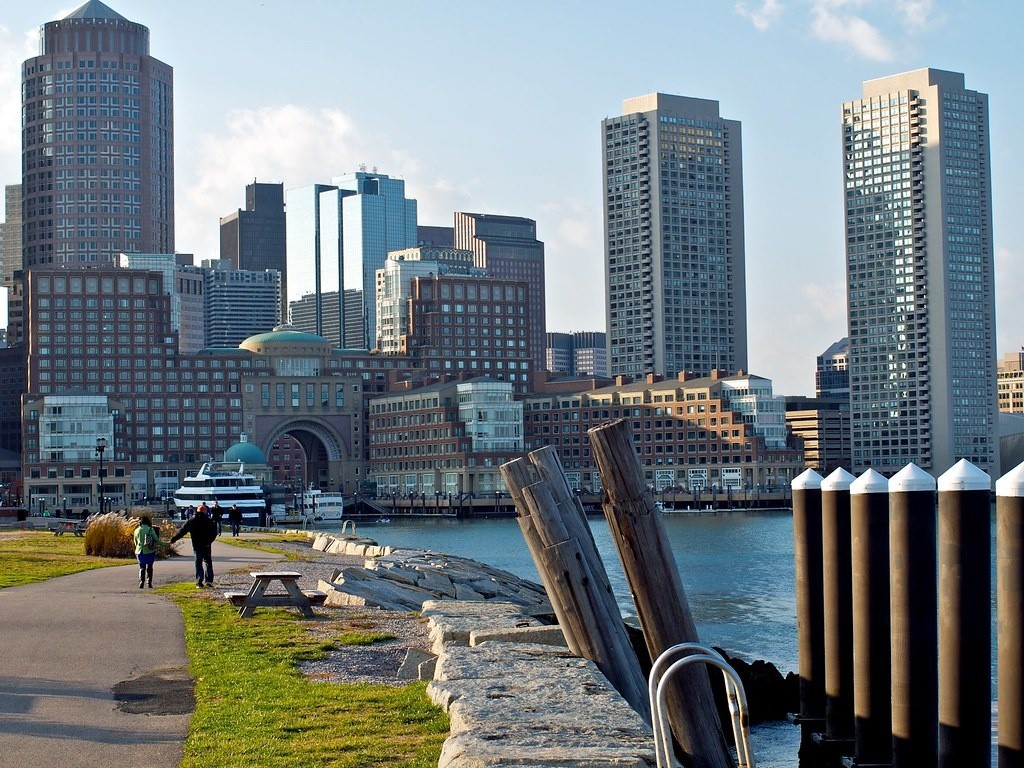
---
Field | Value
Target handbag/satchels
[144,527,157,550]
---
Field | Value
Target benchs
[224,591,251,607]
[49,528,60,532]
[301,589,328,606]
[76,528,86,533]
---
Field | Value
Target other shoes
[196,584,204,589]
[139,582,144,588]
[206,581,213,587]
[147,584,153,588]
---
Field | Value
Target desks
[237,571,315,619]
[54,519,84,537]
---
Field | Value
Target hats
[197,506,206,514]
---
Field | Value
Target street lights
[661,485,665,510]
[392,492,395,513]
[421,491,425,513]
[711,481,793,510]
[42,498,45,513]
[354,492,356,513]
[436,491,439,514]
[410,490,414,513]
[108,496,112,512]
[697,483,701,511]
[672,484,676,511]
[692,484,696,509]
[104,496,107,514]
[95,437,106,515]
[39,498,42,513]
[448,491,452,514]
[63,497,67,519]
[496,491,500,512]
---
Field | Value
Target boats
[263,475,344,520]
[172,450,266,526]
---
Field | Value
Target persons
[167,506,217,589]
[202,502,210,521]
[134,516,171,589]
[43,507,50,517]
[229,504,244,537]
[211,502,223,537]
[185,505,195,521]
